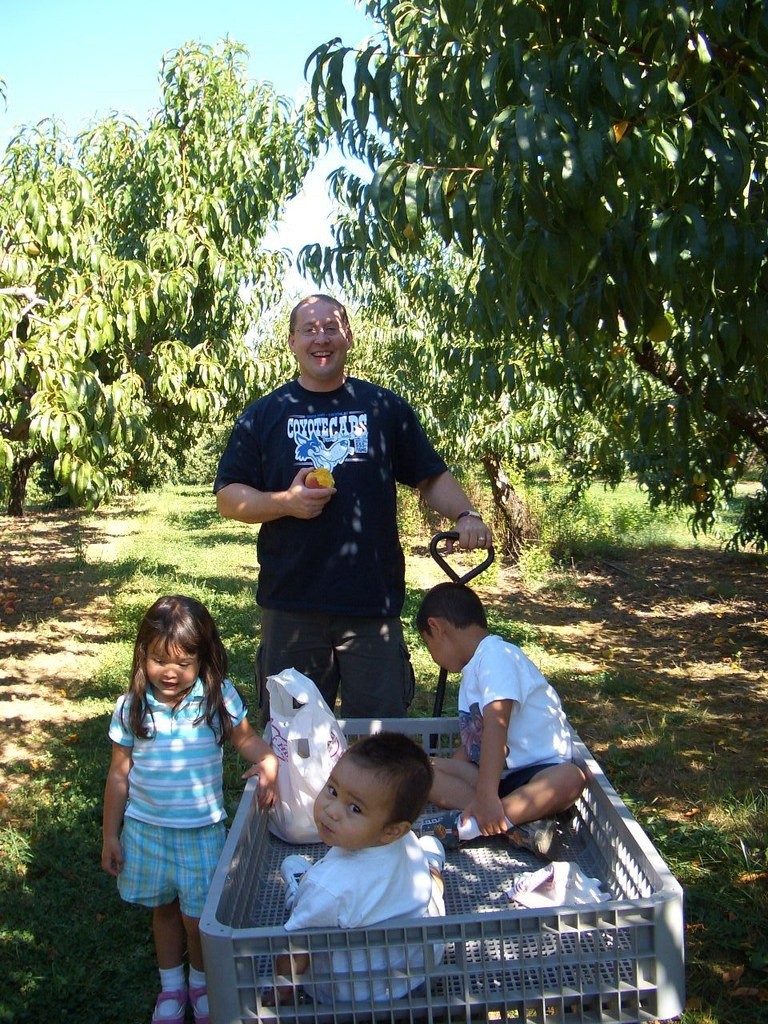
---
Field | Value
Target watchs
[455,509,483,523]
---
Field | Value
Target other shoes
[189,986,211,1024]
[151,989,188,1024]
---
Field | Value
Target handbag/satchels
[266,667,351,846]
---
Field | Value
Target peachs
[642,313,675,341]
[305,468,335,489]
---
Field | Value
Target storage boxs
[197,714,687,1024]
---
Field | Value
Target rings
[477,535,486,542]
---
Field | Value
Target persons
[401,584,587,856]
[263,731,447,1010]
[100,595,285,1024]
[214,293,493,722]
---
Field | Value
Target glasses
[293,326,345,336]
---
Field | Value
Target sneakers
[280,856,313,910]
[410,809,465,850]
[417,835,445,877]
[504,820,556,858]
